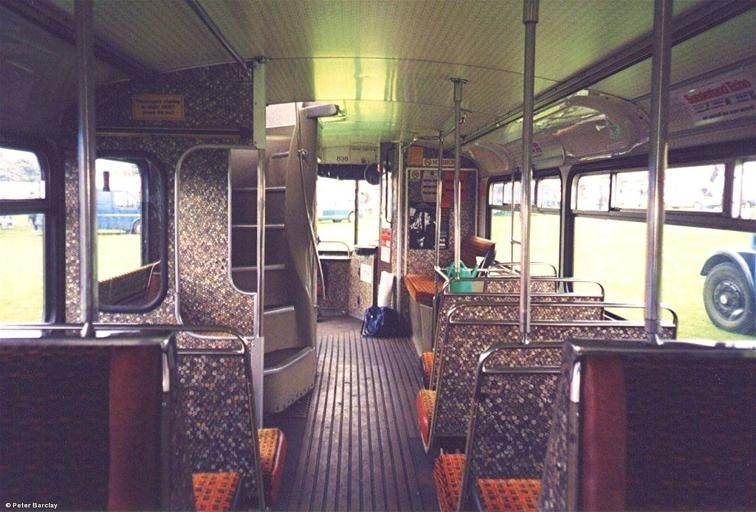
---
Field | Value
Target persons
[407,216,422,248]
[420,213,435,250]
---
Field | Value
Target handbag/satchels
[361,303,412,339]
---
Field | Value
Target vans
[315,175,359,223]
[30,182,143,236]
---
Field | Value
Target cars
[697,231,756,335]
